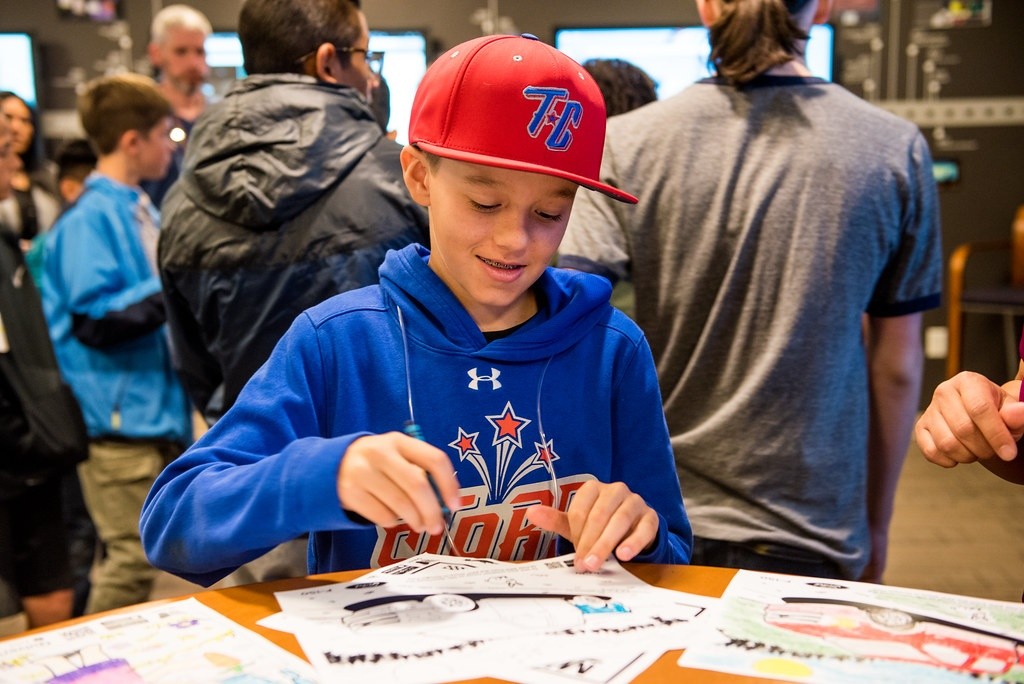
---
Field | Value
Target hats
[409,33,639,204]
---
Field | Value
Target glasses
[296,48,385,75]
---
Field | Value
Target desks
[0,556,1023,683]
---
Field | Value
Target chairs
[948,210,1024,380]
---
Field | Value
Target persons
[915,326,1024,485]
[582,58,658,118]
[139,33,694,590]
[137,3,212,208]
[555,0,943,584]
[155,0,431,431]
[36,73,195,616]
[0,89,99,632]
